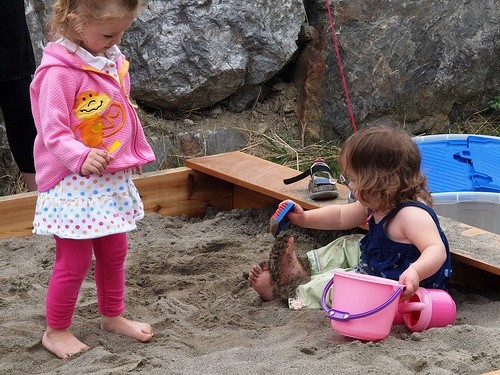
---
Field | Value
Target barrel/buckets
[399,287,456,332]
[321,271,406,341]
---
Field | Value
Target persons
[248,125,452,324]
[0,0,37,192]
[30,0,155,359]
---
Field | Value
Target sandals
[339,173,355,204]
[283,157,339,200]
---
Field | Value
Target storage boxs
[412,134,500,237]
